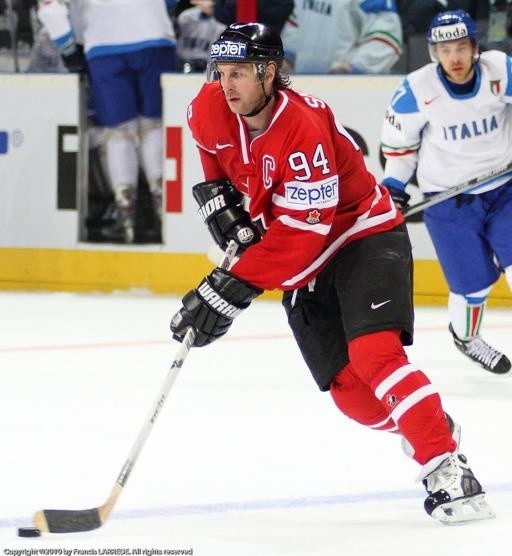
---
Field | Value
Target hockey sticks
[34,239,238,534]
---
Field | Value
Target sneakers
[414,450,481,515]
[84,198,134,229]
[448,322,511,374]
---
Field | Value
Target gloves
[192,176,261,257]
[61,43,91,82]
[170,266,264,347]
[385,184,410,212]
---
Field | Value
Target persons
[171,19,487,518]
[2,0,511,247]
[379,9,512,374]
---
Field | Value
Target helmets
[205,22,284,86]
[426,9,480,63]
